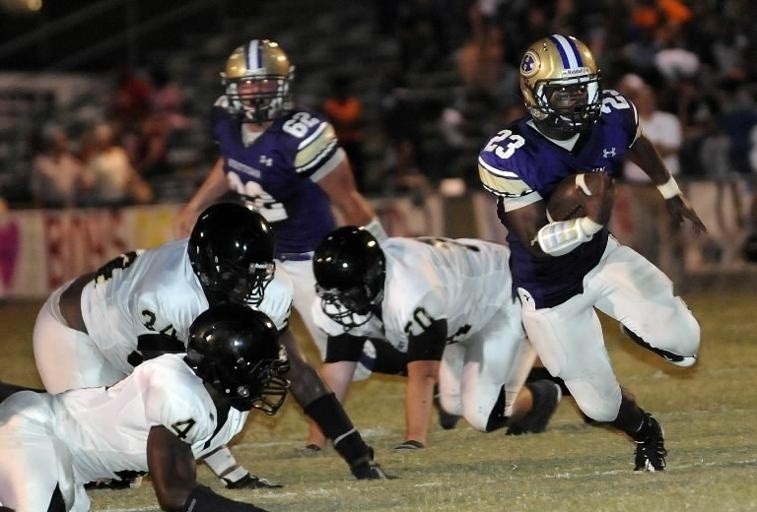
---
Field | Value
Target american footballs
[545,171,603,225]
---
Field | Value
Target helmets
[518,34,600,133]
[226,41,294,124]
[189,201,275,309]
[312,226,384,327]
[185,305,291,413]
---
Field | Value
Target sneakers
[507,381,561,439]
[620,322,697,367]
[635,415,670,472]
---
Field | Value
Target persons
[283,223,563,459]
[0,304,279,512]
[0,0,756,213]
[477,34,708,475]
[1,199,401,491]
[173,37,461,430]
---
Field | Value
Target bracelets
[360,216,390,246]
[300,393,354,441]
[181,483,254,512]
[202,447,235,476]
